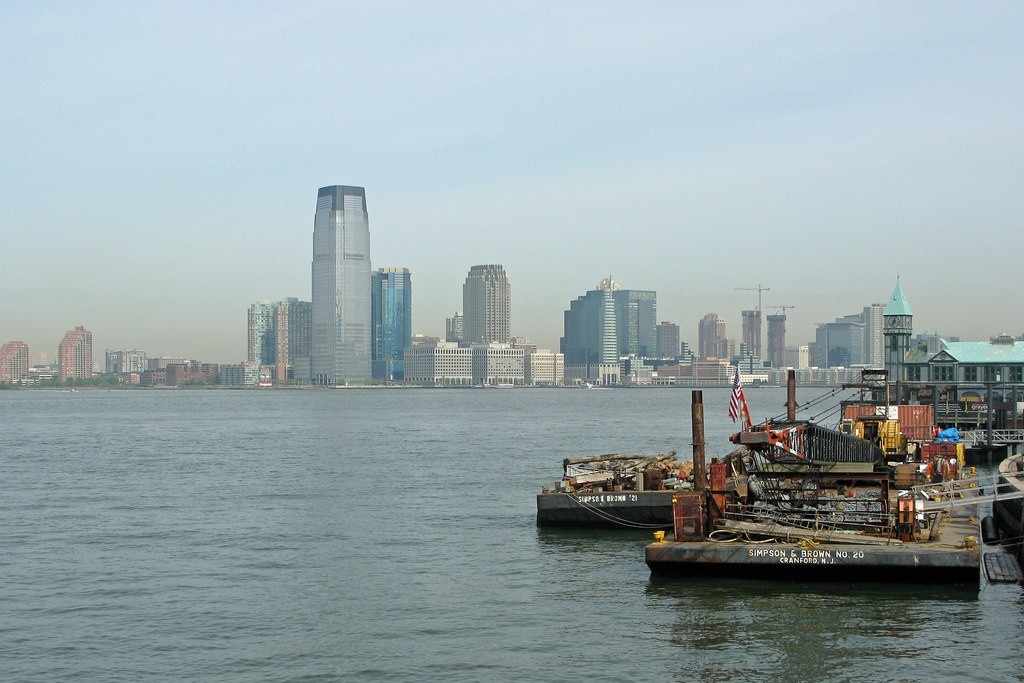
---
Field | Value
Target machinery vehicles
[816,412,920,491]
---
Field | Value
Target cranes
[735,284,771,312]
[767,306,795,319]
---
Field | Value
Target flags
[728,360,752,427]
